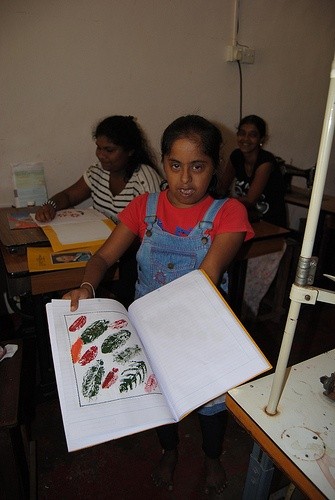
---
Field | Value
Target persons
[63,114,256,494]
[34,116,165,251]
[220,115,280,224]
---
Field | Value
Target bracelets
[80,281,96,299]
[41,201,58,209]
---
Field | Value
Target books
[44,270,274,453]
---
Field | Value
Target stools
[258,238,298,317]
[0,338,40,500]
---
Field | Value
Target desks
[228,218,292,320]
[0,205,140,406]
